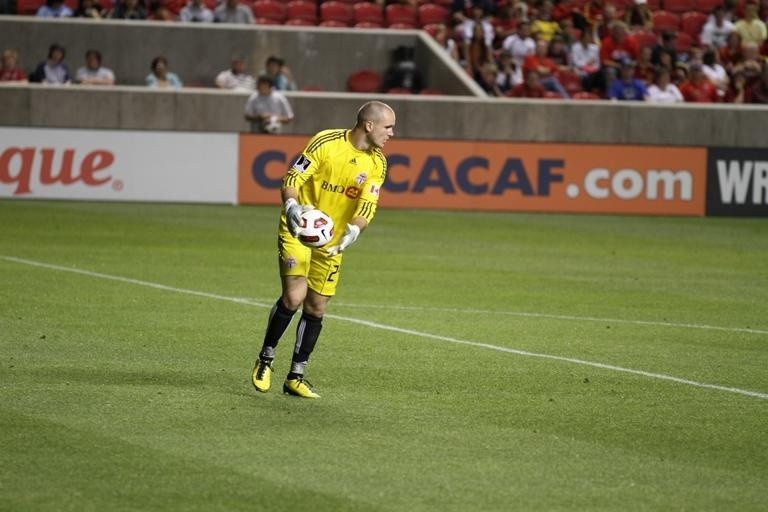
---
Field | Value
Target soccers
[265,115,284,136]
[295,208,335,250]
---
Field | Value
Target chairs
[493,1,767,104]
[17,1,448,41]
[302,69,448,96]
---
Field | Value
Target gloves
[283,197,316,239]
[326,222,360,258]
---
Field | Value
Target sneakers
[251,350,274,392]
[282,377,325,401]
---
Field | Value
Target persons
[240,75,296,134]
[250,99,398,402]
